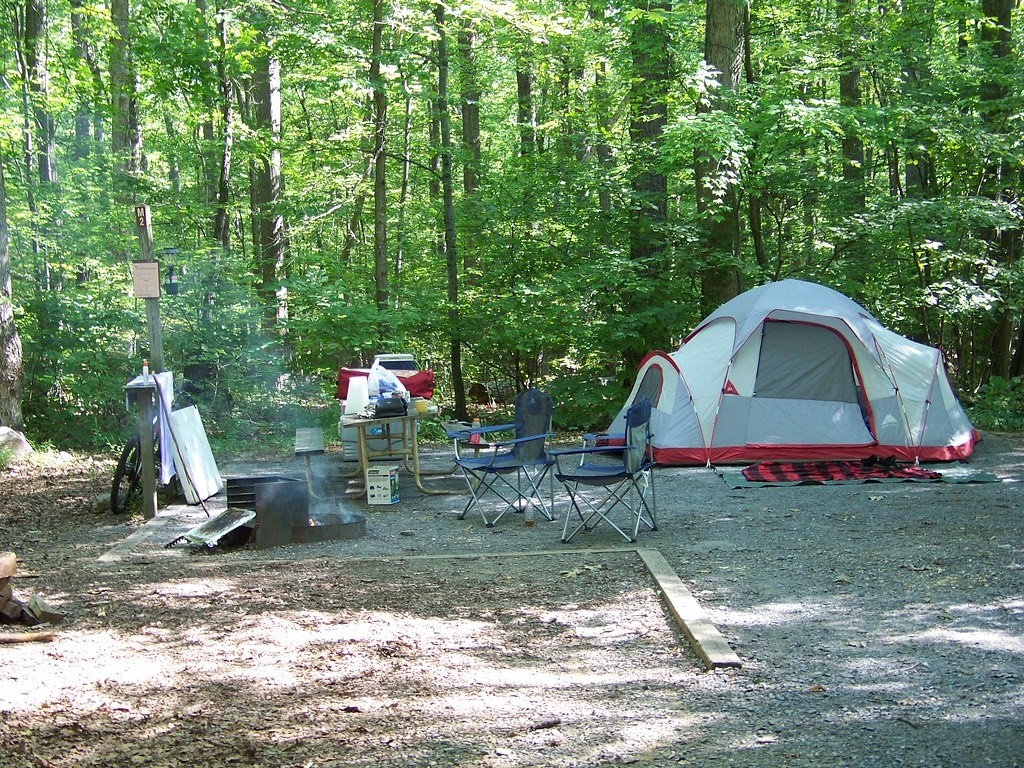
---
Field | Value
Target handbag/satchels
[335,356,434,417]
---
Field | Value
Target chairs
[549,396,658,545]
[448,388,558,528]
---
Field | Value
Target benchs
[294,427,344,500]
[439,423,491,495]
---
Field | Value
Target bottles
[470,418,481,444]
[525,497,535,530]
[142,361,149,385]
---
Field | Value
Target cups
[415,400,428,413]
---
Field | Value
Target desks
[341,404,458,500]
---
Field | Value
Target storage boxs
[366,466,401,505]
[374,354,415,370]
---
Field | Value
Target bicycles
[110,368,199,517]
